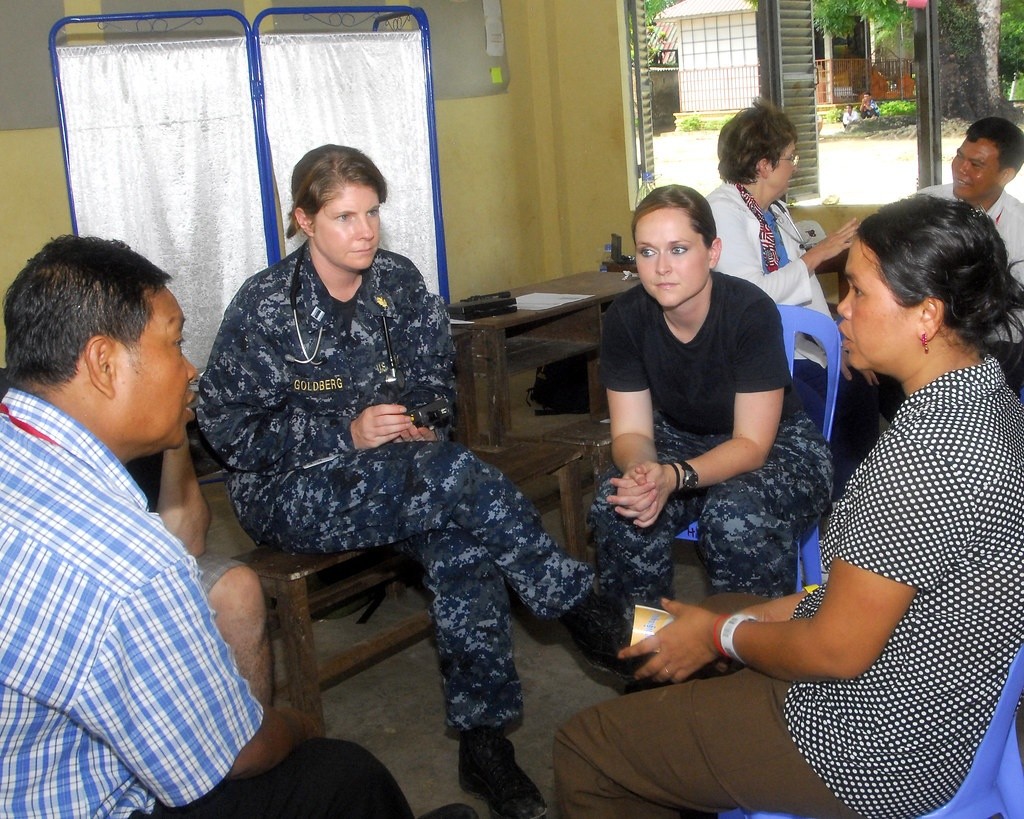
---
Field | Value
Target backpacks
[524,353,590,416]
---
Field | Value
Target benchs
[230,411,614,742]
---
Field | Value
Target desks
[190,272,644,477]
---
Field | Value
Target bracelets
[668,463,680,491]
[803,584,819,595]
[713,614,756,664]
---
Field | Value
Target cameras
[408,398,453,430]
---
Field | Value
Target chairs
[675,305,842,595]
[719,641,1024,819]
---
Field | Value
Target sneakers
[559,593,673,689]
[458,724,548,819]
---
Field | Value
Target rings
[664,667,672,676]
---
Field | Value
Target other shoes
[414,803,479,819]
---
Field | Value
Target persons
[0,233,481,819]
[704,102,879,496]
[817,92,879,136]
[198,144,655,819]
[556,195,1024,818]
[587,186,833,676]
[920,117,1024,341]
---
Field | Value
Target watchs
[676,461,699,490]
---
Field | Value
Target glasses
[775,153,800,166]
